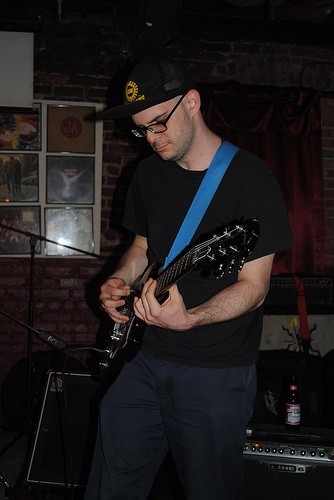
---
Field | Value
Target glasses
[130,92,188,138]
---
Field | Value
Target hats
[105,60,191,114]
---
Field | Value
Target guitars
[98,214,261,370]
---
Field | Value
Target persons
[0,215,41,245]
[4,156,22,194]
[82,57,293,500]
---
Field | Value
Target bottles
[285,374,303,433]
